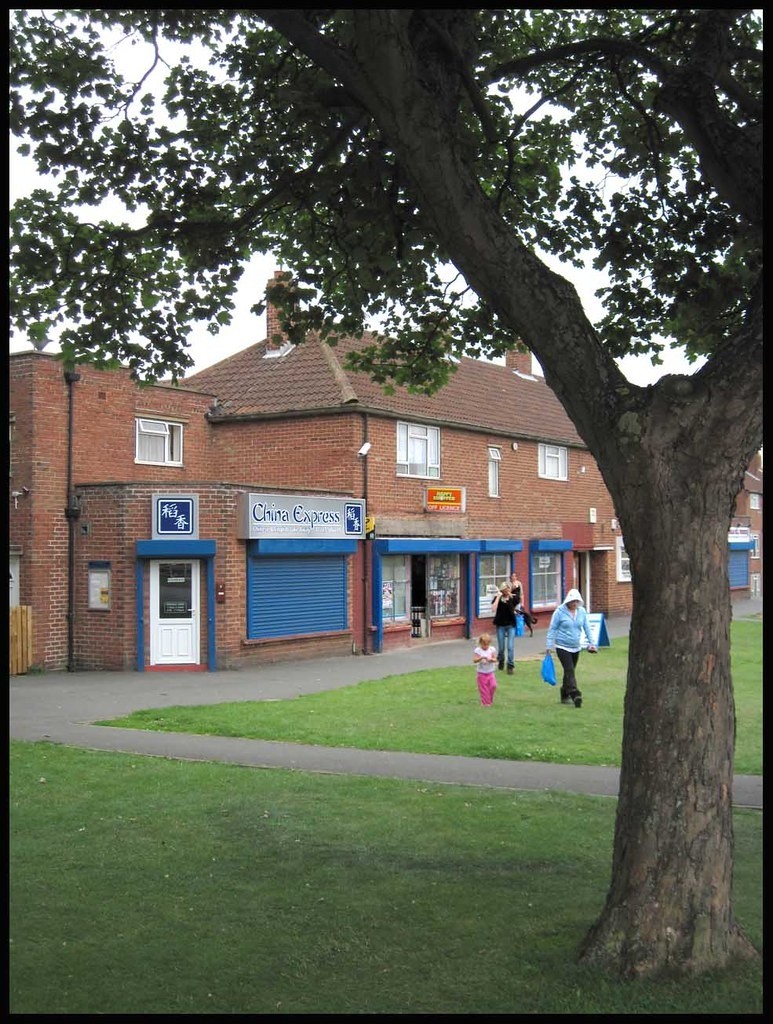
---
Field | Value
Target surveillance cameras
[357,442,371,456]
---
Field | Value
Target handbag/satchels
[541,653,557,686]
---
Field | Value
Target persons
[510,572,525,637]
[491,581,516,675]
[546,589,597,708]
[473,632,499,707]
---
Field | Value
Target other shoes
[508,664,513,674]
[498,661,504,670]
[561,687,569,703]
[574,696,583,707]
